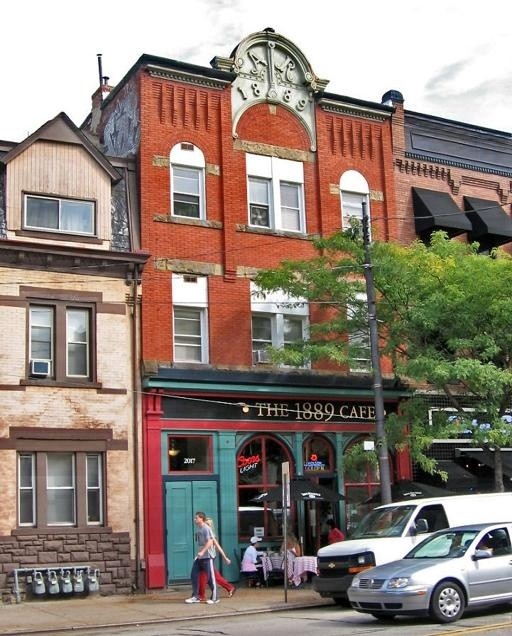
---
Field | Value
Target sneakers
[206,599,221,604]
[228,586,235,597]
[197,595,207,601]
[185,597,200,603]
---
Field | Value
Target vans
[312,492,512,608]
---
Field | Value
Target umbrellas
[356,475,460,503]
[247,474,349,555]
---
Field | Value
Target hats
[251,537,263,544]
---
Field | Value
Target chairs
[233,548,285,592]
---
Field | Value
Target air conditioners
[30,360,51,378]
[253,350,273,365]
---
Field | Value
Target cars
[347,520,512,622]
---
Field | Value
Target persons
[280,525,301,557]
[474,532,493,557]
[185,511,220,603]
[326,518,345,544]
[198,519,237,601]
[242,537,266,589]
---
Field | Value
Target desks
[261,556,318,592]
